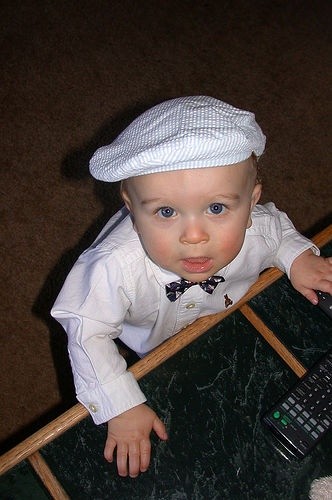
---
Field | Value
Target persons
[51,96,332,477]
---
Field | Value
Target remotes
[261,347,332,461]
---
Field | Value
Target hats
[89,96,266,182]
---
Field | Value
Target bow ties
[166,276,225,302]
